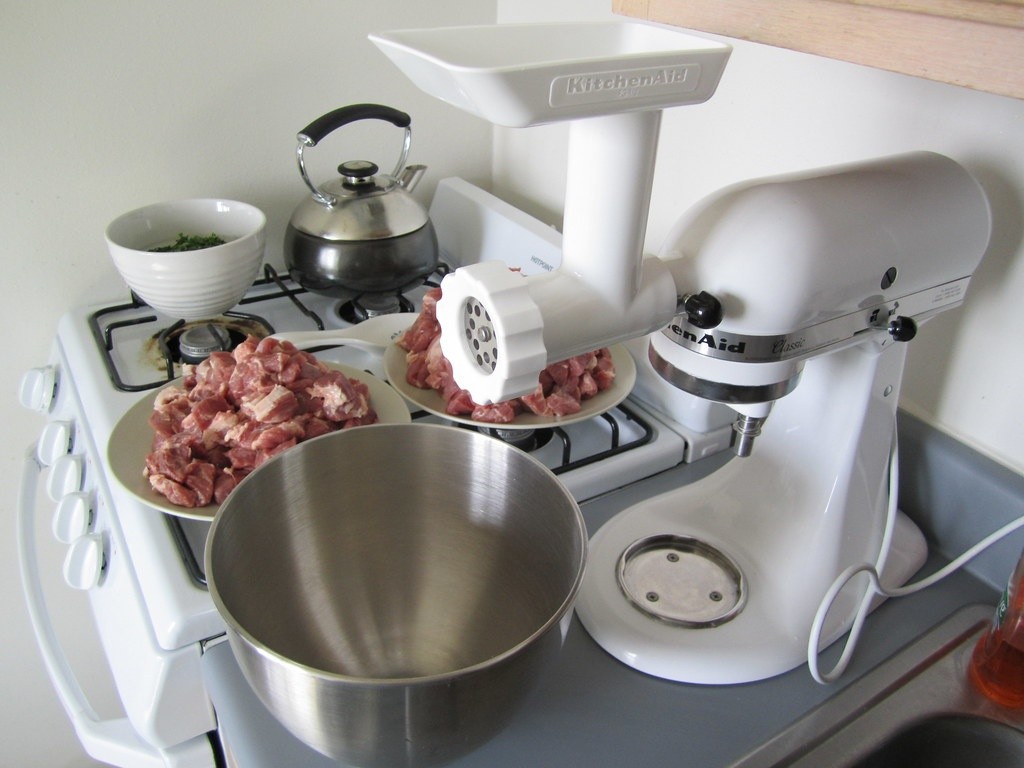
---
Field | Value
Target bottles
[970,538,1024,714]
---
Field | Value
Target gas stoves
[19,177,736,751]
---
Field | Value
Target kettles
[285,104,438,300]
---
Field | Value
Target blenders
[366,15,990,684]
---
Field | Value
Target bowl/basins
[203,424,588,767]
[105,198,267,322]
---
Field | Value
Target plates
[106,359,411,523]
[384,332,637,428]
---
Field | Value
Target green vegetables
[145,232,227,253]
